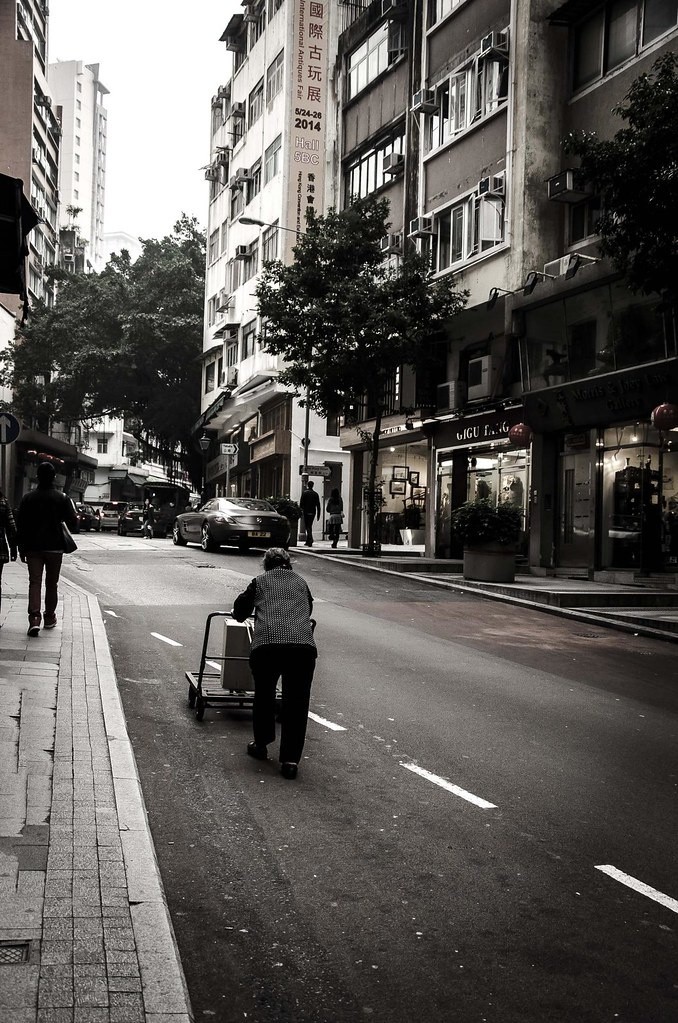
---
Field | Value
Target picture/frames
[390,480,406,494]
[392,466,408,480]
[409,471,419,486]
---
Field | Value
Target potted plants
[397,505,427,545]
[446,495,528,585]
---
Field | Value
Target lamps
[564,252,602,282]
[522,271,557,297]
[405,417,440,430]
[494,400,516,412]
[454,406,476,418]
[486,287,516,312]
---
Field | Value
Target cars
[172,497,291,554]
[75,501,158,536]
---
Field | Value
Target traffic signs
[220,443,239,455]
[299,465,332,477]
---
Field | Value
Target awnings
[128,475,147,485]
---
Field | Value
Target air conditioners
[229,176,240,185]
[214,294,228,312]
[547,170,594,203]
[477,176,506,196]
[383,153,404,172]
[64,254,74,262]
[221,367,236,385]
[381,234,399,249]
[412,89,435,108]
[236,245,249,259]
[380,0,404,18]
[236,168,248,179]
[409,216,432,236]
[205,152,227,181]
[211,84,244,119]
[481,30,507,54]
[542,254,601,282]
[44,96,52,105]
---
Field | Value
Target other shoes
[247,741,268,761]
[304,543,312,547]
[281,764,299,780]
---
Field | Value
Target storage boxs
[220,618,256,689]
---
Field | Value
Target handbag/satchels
[59,522,77,554]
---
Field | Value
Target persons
[326,488,345,548]
[18,461,78,638]
[244,492,250,498]
[95,509,100,516]
[300,481,320,547]
[142,498,154,539]
[0,487,18,627]
[231,547,318,780]
[185,502,193,512]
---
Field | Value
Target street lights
[199,431,210,508]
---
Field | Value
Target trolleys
[185,611,317,720]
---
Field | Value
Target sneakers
[27,617,40,637]
[43,612,57,629]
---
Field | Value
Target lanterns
[507,422,533,449]
[650,401,678,433]
